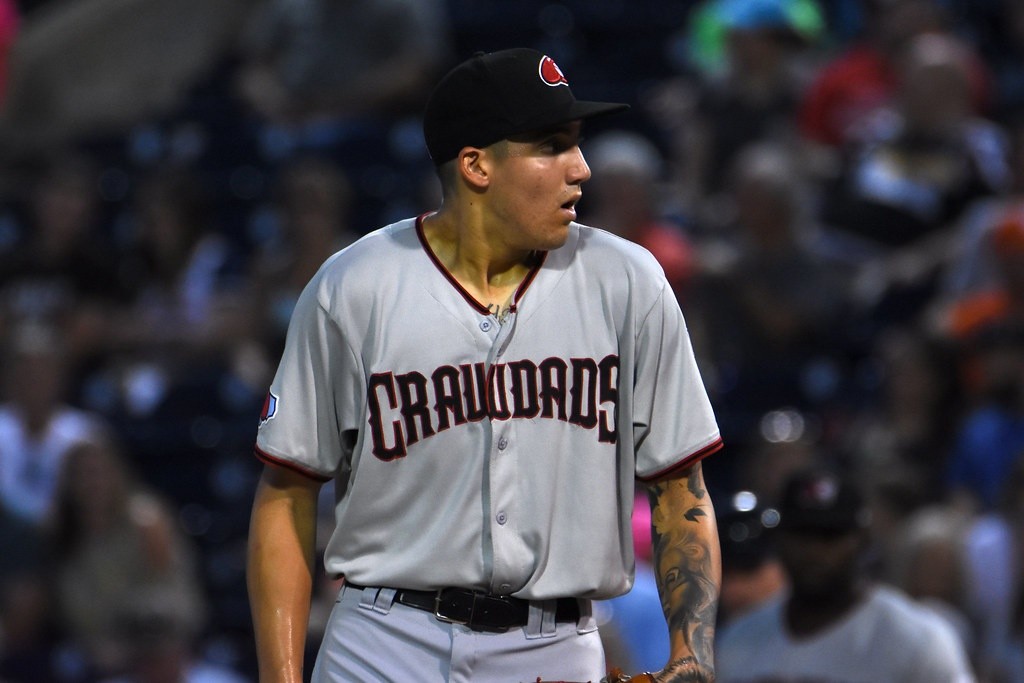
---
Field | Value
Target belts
[344,579,591,633]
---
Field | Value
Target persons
[243,48,722,683]
[0,0,1024,683]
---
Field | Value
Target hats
[423,48,631,160]
[764,470,867,540]
